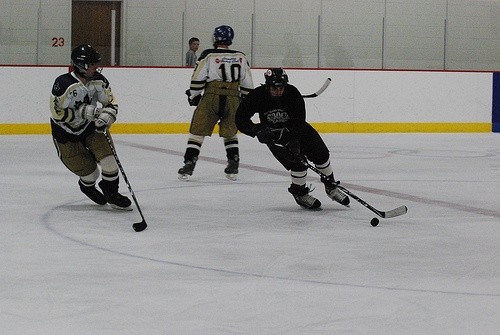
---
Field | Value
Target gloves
[95,108,116,133]
[80,104,97,121]
[254,123,275,147]
[287,143,300,161]
[185,89,202,106]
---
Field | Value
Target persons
[186,37,200,66]
[178,25,254,176]
[235,67,350,209]
[49,44,131,208]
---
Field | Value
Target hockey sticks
[94,115,148,233]
[301,78,332,98]
[294,150,408,219]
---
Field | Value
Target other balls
[370,218,379,226]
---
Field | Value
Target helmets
[264,68,289,87]
[214,25,234,45]
[71,44,102,78]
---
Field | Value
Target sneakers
[224,155,239,181]
[104,192,134,211]
[177,157,198,182]
[325,183,350,208]
[78,180,107,206]
[289,184,323,210]
